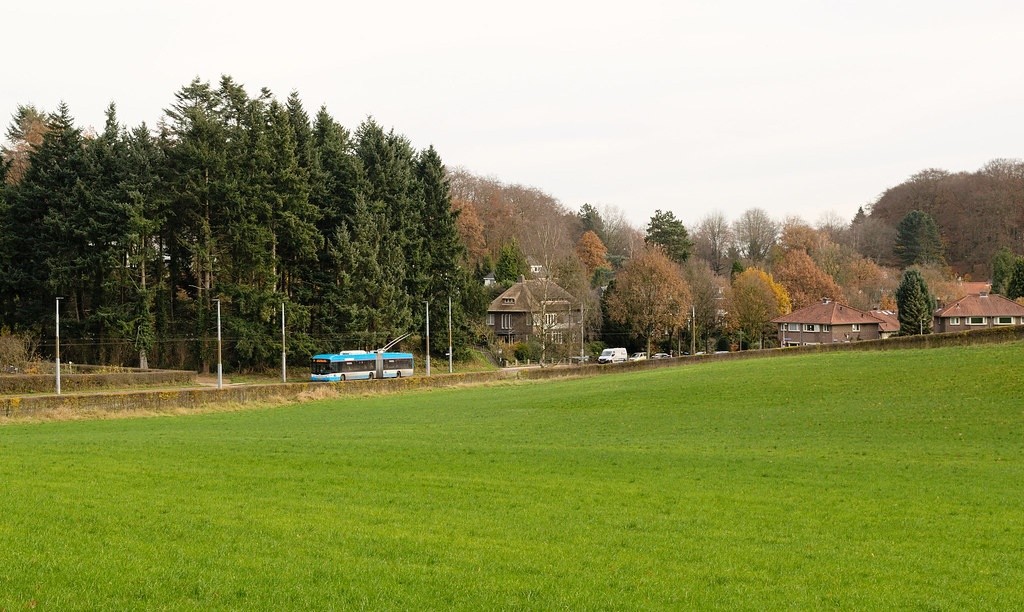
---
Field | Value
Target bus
[309,332,418,383]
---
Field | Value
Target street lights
[55,296,64,396]
[212,298,224,390]
[421,300,434,378]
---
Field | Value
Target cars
[651,352,670,359]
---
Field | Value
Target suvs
[629,352,647,361]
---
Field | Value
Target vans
[598,348,628,364]
